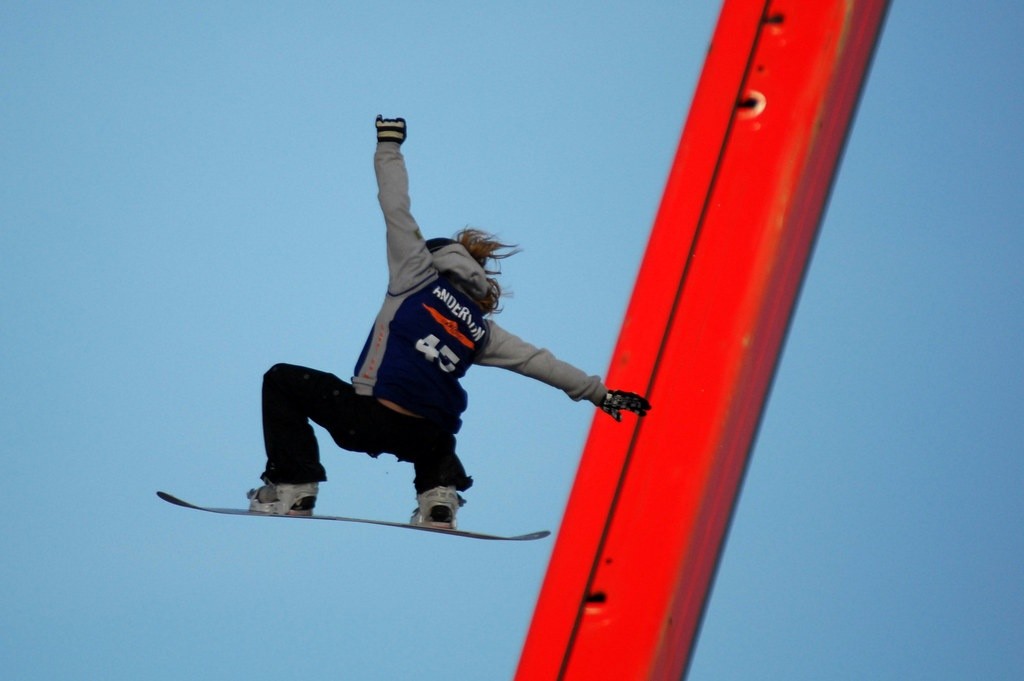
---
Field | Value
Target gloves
[375,114,407,144]
[598,389,650,422]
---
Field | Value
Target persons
[246,114,651,533]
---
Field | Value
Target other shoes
[245,480,318,517]
[411,486,459,528]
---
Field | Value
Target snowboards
[155,487,553,542]
